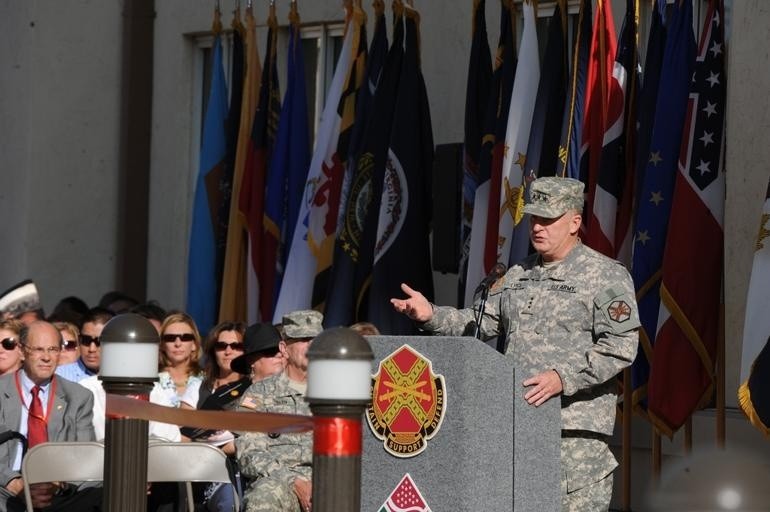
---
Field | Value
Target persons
[349,322,380,336]
[0,277,324,512]
[390,175,642,512]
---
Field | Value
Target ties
[28,386,48,448]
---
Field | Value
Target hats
[282,310,325,338]
[521,177,586,218]
[230,322,282,375]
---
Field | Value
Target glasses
[164,332,195,343]
[1,337,21,351]
[214,341,243,350]
[81,333,103,346]
[25,343,58,353]
[58,339,79,351]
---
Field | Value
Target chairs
[146,442,240,512]
[21,442,105,512]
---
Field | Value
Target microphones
[474,262,506,294]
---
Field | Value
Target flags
[458,0,730,436]
[178,3,434,356]
[736,177,769,447]
